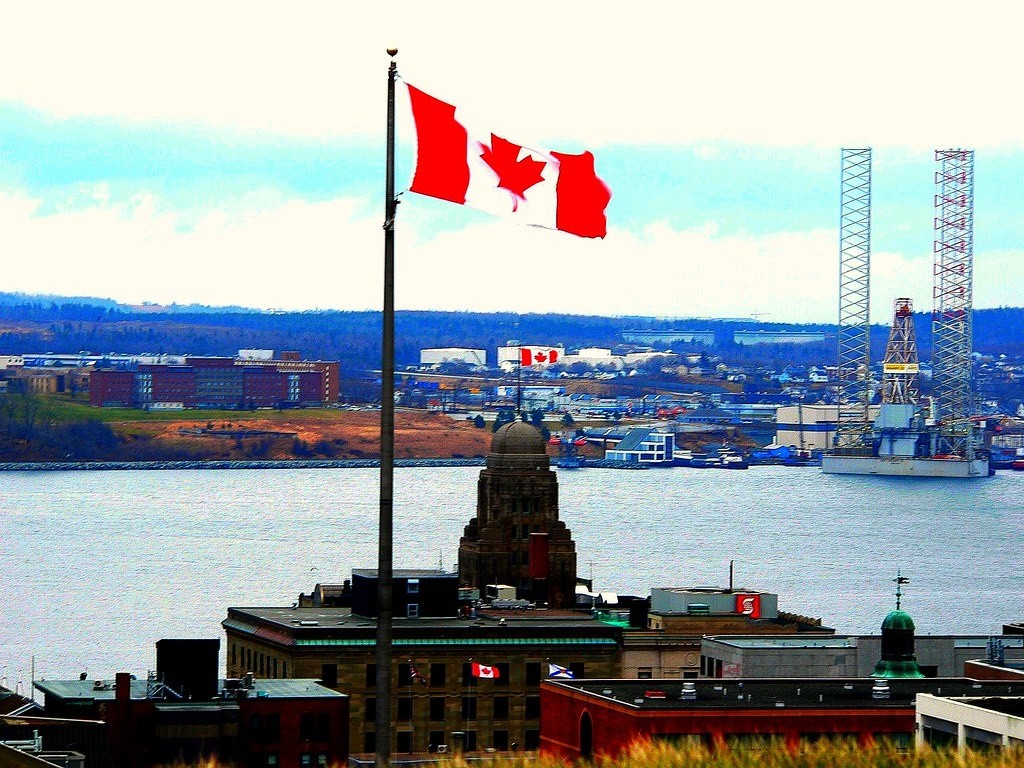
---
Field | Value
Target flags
[519,347,561,366]
[410,664,428,688]
[548,662,575,679]
[472,662,501,679]
[396,74,613,241]
